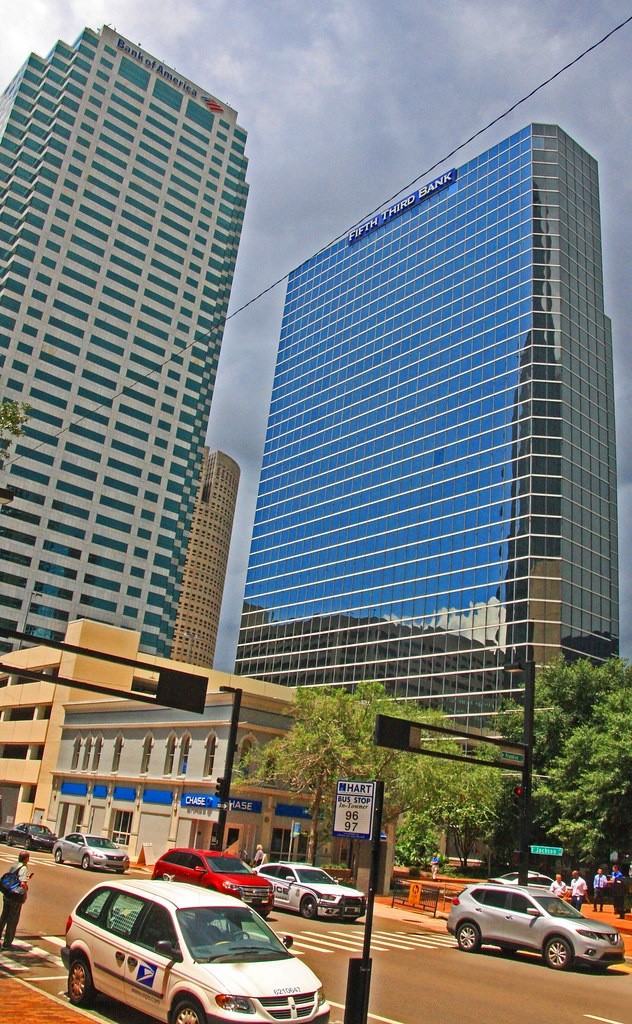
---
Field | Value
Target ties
[598,876,600,887]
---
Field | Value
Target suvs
[445,882,626,971]
[150,848,275,919]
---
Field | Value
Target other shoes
[593,909,597,912]
[600,909,602,912]
[616,917,624,919]
[1,944,17,950]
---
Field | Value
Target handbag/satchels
[0,865,28,899]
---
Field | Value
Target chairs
[149,914,224,947]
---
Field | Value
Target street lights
[212,683,244,852]
[503,660,537,886]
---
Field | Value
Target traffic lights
[514,786,523,796]
[215,777,224,797]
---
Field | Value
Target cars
[487,869,572,894]
[5,822,59,851]
[249,860,366,924]
[51,832,130,874]
[60,879,331,1024]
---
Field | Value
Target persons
[607,865,623,914]
[254,844,263,866]
[550,874,567,898]
[431,853,440,880]
[566,871,590,912]
[0,851,34,950]
[612,878,626,919]
[593,869,607,912]
